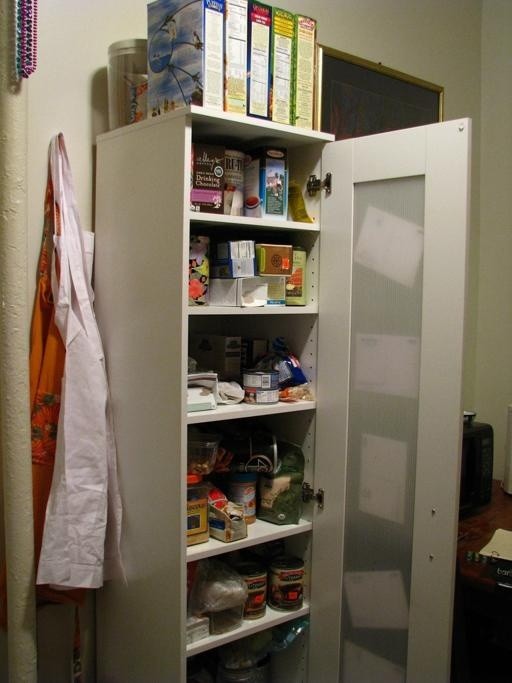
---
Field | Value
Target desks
[455,478,512,682]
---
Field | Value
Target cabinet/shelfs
[96,106,473,683]
